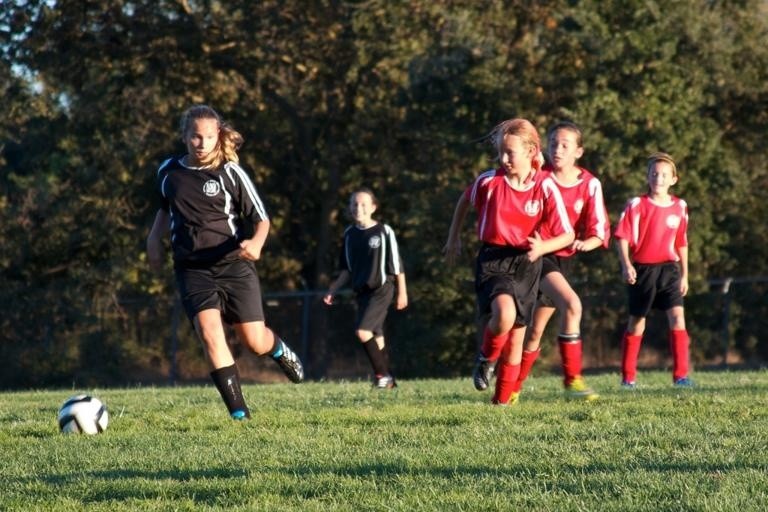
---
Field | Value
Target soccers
[58,395,109,436]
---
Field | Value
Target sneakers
[674,377,694,386]
[230,408,252,422]
[269,334,304,384]
[472,350,498,392]
[494,392,520,407]
[565,374,601,404]
[620,380,636,387]
[373,369,394,389]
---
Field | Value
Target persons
[323,186,409,389]
[612,151,703,388]
[506,121,613,405]
[146,106,305,420]
[440,118,576,405]
